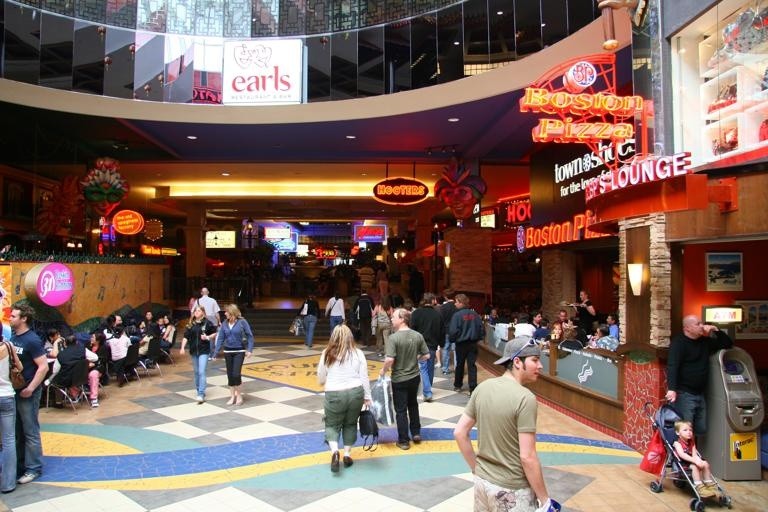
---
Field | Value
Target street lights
[431,223,445,294]
[239,216,263,308]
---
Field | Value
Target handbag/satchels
[300,299,309,316]
[288,316,305,336]
[360,405,379,436]
[3,341,26,389]
[371,374,397,426]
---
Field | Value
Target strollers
[642,400,732,512]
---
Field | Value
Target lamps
[627,263,643,296]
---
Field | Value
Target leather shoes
[331,451,340,472]
[344,455,353,464]
[48,401,63,408]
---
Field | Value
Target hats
[493,335,541,365]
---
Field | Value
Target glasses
[511,338,538,361]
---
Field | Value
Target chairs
[46,317,179,415]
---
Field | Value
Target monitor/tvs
[204,230,241,251]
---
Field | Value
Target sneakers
[706,484,716,494]
[17,469,40,483]
[454,386,462,392]
[413,434,421,441]
[396,440,410,449]
[698,486,715,498]
[91,399,100,407]
[434,363,442,368]
[423,395,432,402]
[195,395,203,403]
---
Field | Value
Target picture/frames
[735,300,768,340]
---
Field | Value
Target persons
[211,303,254,406]
[191,286,223,362]
[188,295,200,314]
[380,308,431,450]
[672,420,718,496]
[179,305,218,403]
[318,260,620,400]
[298,292,321,347]
[665,315,733,458]
[0,300,177,493]
[454,335,562,512]
[317,324,373,472]
